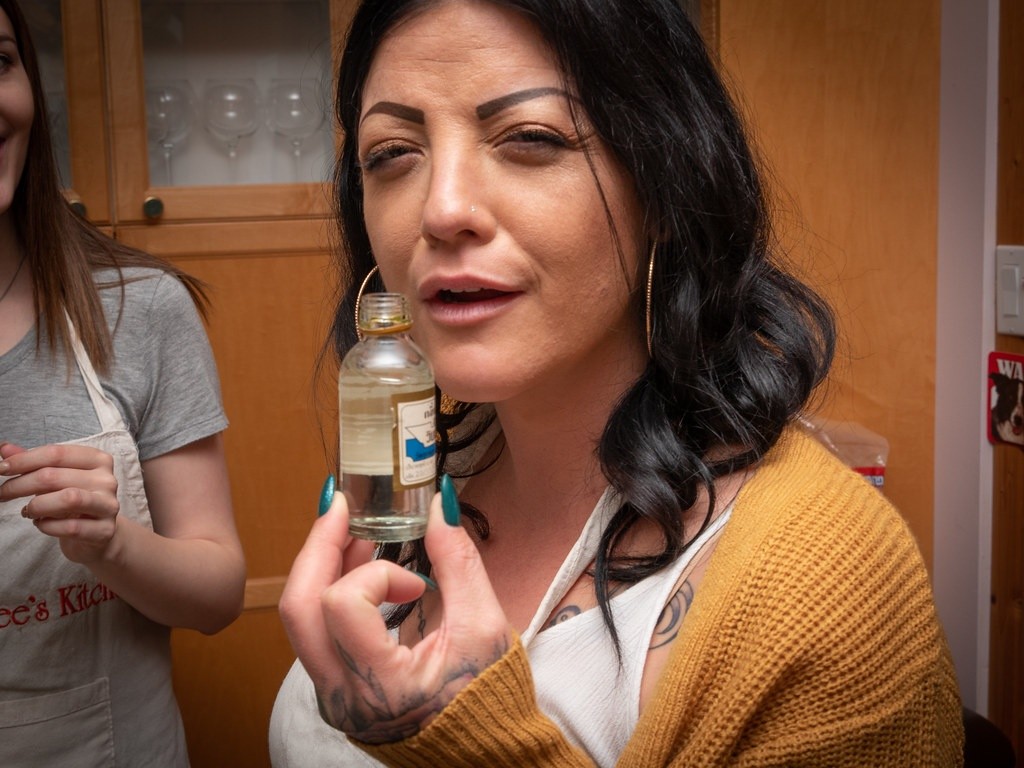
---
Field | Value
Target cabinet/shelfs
[7,1,404,607]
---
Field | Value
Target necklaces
[0,252,29,302]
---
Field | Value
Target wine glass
[146,74,198,185]
[200,77,260,184]
[265,77,325,182]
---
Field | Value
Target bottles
[335,290,436,544]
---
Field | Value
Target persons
[0,0,251,768]
[267,0,966,768]
